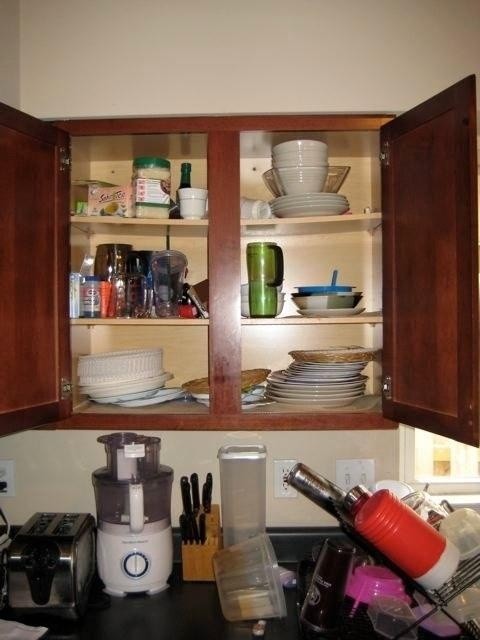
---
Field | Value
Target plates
[264,348,376,407]
[76,347,189,408]
[267,192,350,217]
[182,369,270,407]
[290,286,367,317]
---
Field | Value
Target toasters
[6,511,103,622]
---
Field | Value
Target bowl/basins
[263,165,278,197]
[241,293,286,302]
[324,165,351,192]
[241,302,284,317]
[270,139,329,196]
[240,282,283,295]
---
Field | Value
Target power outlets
[0,460,16,497]
[274,460,298,497]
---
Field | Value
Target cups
[148,250,188,317]
[299,537,353,637]
[247,241,284,317]
[177,187,209,220]
[353,489,462,589]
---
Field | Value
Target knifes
[179,472,215,545]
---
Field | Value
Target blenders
[90,433,175,598]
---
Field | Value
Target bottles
[176,163,191,205]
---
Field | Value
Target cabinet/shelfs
[0,73,480,449]
